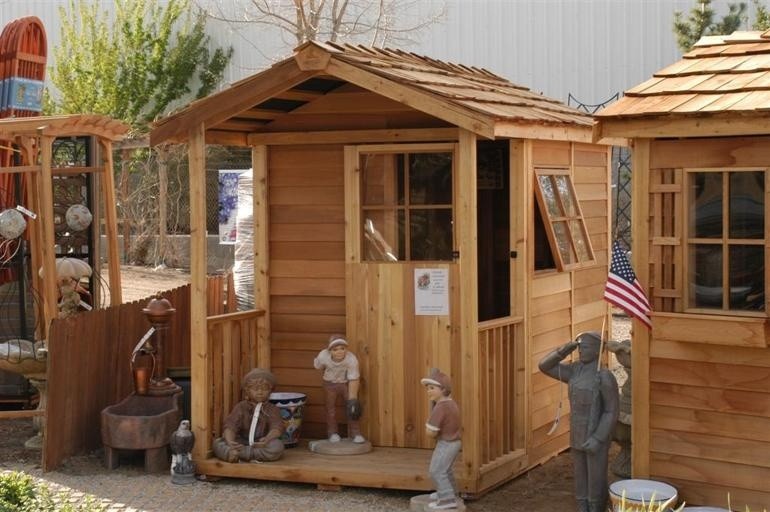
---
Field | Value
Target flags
[603,239,653,330]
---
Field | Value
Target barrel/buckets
[268,391,306,449]
[130,349,156,398]
[609,479,680,511]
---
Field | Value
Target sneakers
[328,434,342,443]
[327,335,350,350]
[352,435,366,444]
[428,499,459,510]
[428,492,440,500]
[242,367,276,388]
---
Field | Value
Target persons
[420,371,462,509]
[213,368,283,462]
[313,334,367,445]
[538,330,622,511]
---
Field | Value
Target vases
[607,477,732,511]
[267,390,309,450]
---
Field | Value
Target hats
[420,372,452,395]
[575,331,606,347]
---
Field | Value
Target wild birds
[169,417,195,465]
